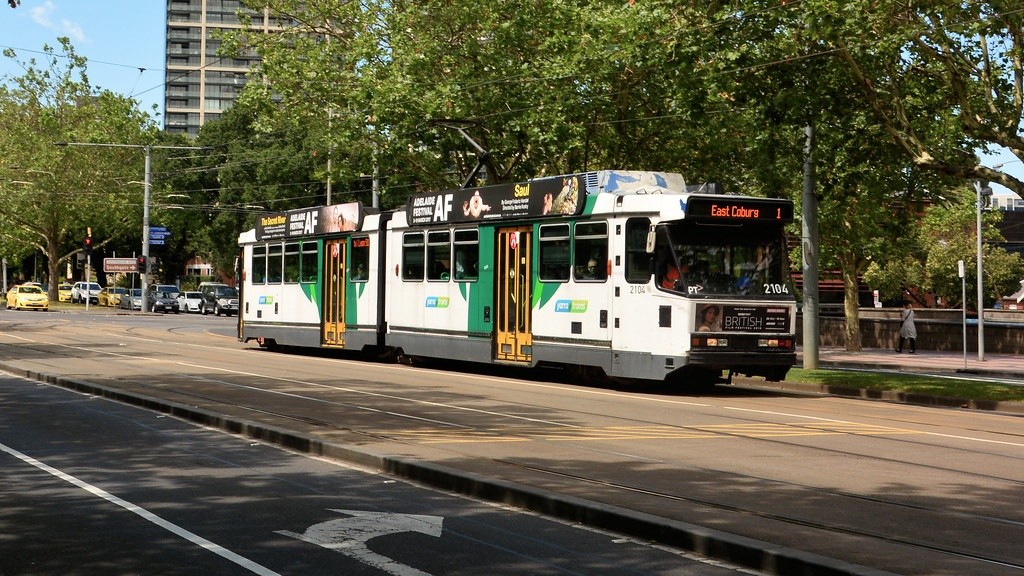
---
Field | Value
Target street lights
[53,140,215,320]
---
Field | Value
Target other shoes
[909,350,914,353]
[895,348,902,353]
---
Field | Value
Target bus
[233,170,798,396]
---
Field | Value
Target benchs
[255,260,568,283]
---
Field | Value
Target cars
[5,284,50,312]
[58,284,74,303]
[120,288,142,311]
[148,290,180,315]
[97,287,125,307]
[176,290,207,313]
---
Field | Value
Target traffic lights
[83,235,94,255]
[137,256,148,274]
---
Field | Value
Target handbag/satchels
[900,321,904,328]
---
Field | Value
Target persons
[895,303,918,353]
[325,206,357,232]
[573,254,689,291]
[262,261,367,283]
[699,305,722,332]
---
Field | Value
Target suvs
[148,283,180,302]
[71,281,103,305]
[197,284,239,318]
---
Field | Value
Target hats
[701,304,720,316]
[359,264,367,272]
